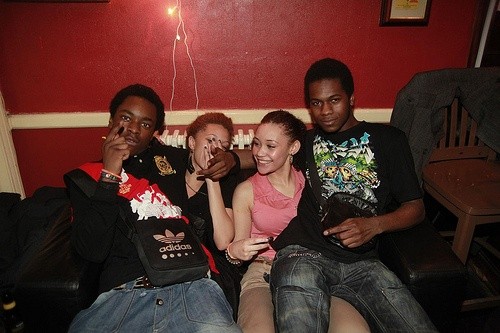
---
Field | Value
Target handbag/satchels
[320,192,377,252]
[133,218,209,286]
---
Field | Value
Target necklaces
[185,182,197,193]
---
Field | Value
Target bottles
[2,291,26,333]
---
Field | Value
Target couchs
[10,169,466,333]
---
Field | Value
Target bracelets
[226,149,240,169]
[225,250,242,264]
[101,168,122,182]
[227,243,237,259]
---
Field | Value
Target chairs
[393,66,500,312]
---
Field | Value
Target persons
[148,113,243,303]
[269,58,438,333]
[225,111,370,333]
[63,83,256,333]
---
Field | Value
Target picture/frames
[380,0,432,28]
